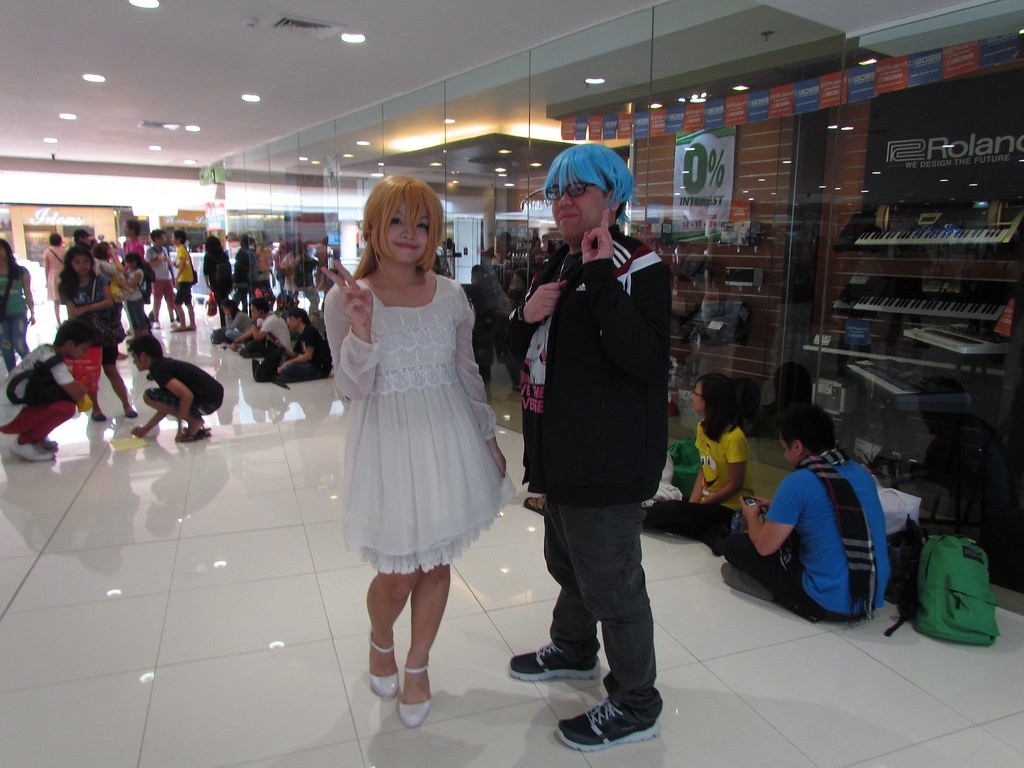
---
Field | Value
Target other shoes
[9,407,212,462]
[721,561,774,601]
[701,523,729,556]
[124,310,196,335]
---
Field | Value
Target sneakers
[510,640,601,682]
[556,696,660,752]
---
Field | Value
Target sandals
[524,496,545,515]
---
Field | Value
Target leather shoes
[369,627,399,698]
[399,660,431,728]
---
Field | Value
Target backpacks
[134,270,150,304]
[253,347,285,382]
[206,252,232,291]
[6,356,73,405]
[900,534,1000,646]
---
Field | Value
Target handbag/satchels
[142,262,154,282]
[208,291,217,316]
[62,345,102,391]
[210,330,225,344]
[0,297,6,321]
[88,275,125,347]
[888,516,928,603]
[192,271,198,284]
[669,436,700,500]
[871,474,921,541]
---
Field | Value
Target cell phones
[260,309,263,315]
[743,496,756,506]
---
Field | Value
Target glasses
[546,181,609,200]
[692,391,703,398]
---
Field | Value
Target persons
[725,403,890,626]
[522,373,756,541]
[0,239,35,375]
[211,299,332,384]
[43,219,327,360]
[509,143,667,752]
[235,234,340,312]
[130,335,224,443]
[656,154,770,401]
[320,174,506,729]
[461,231,529,392]
[0,320,93,462]
[57,245,138,421]
